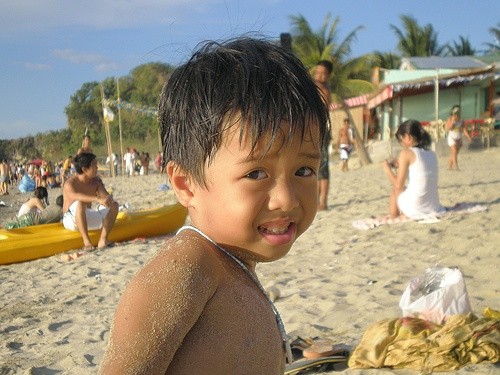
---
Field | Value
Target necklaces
[176,225,298,365]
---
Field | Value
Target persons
[78,135,94,155]
[17,186,47,222]
[9,148,164,188]
[339,119,353,171]
[445,105,471,170]
[490,91,500,146]
[56,195,64,218]
[315,59,333,211]
[380,120,444,220]
[99,35,331,375]
[0,159,12,195]
[62,152,119,249]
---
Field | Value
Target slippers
[283,355,347,375]
[303,342,350,359]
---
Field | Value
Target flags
[102,96,114,122]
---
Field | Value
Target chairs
[386,117,496,153]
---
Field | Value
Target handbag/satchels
[398,267,472,325]
